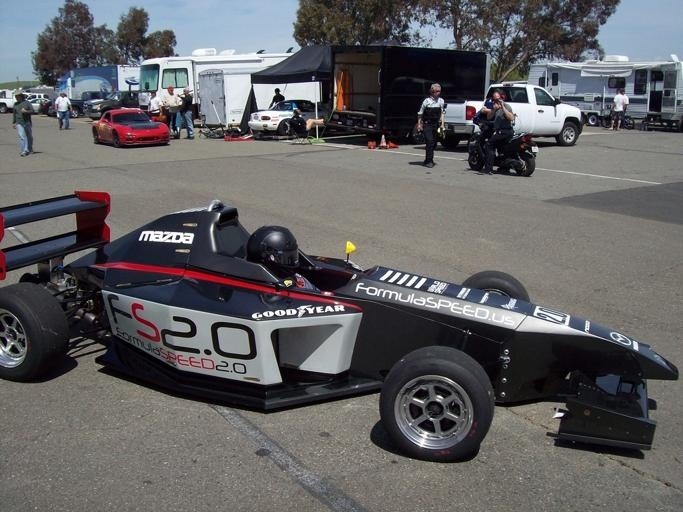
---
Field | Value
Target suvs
[83,90,139,120]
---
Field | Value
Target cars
[0,89,52,114]
[92,108,170,147]
[248,100,328,136]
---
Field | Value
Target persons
[147,90,163,117]
[290,109,324,133]
[472,91,506,147]
[246,224,325,292]
[54,92,74,129]
[607,88,629,131]
[11,90,33,158]
[481,100,516,175]
[161,85,182,139]
[176,89,194,140]
[416,82,446,167]
[268,88,286,108]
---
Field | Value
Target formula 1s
[0,192,678,463]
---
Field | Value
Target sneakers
[21,152,30,157]
[425,162,434,168]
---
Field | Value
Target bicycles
[601,105,635,130]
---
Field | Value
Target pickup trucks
[440,82,588,146]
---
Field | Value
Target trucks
[49,65,141,118]
[137,47,320,130]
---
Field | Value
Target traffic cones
[377,134,387,149]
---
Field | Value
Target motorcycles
[468,123,539,175]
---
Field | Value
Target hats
[13,90,28,98]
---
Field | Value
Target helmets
[247,225,300,278]
